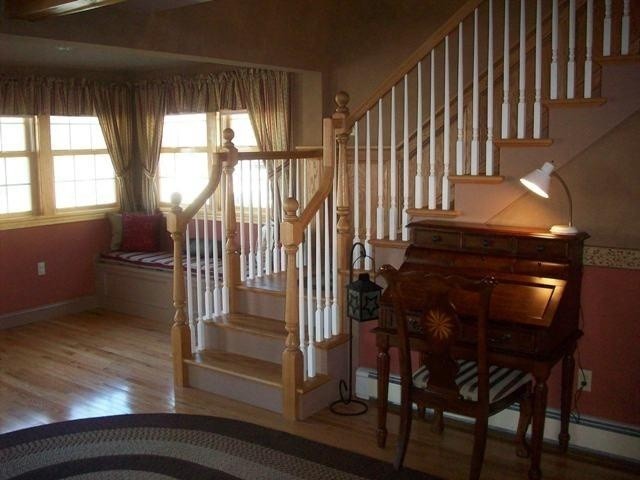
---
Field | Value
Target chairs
[377,263,538,480]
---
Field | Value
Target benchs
[95,248,265,327]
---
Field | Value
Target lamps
[328,242,385,416]
[519,161,581,235]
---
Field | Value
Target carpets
[0,414,451,480]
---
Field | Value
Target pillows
[105,212,164,252]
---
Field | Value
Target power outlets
[577,367,593,393]
